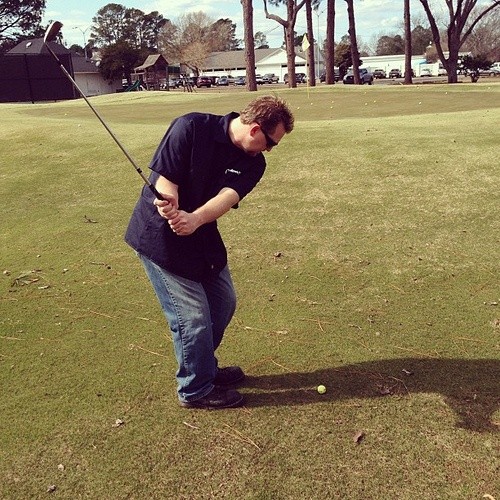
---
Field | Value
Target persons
[123,95,294,410]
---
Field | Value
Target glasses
[254,120,278,148]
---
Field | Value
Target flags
[302,33,310,51]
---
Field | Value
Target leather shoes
[180,385,244,409]
[213,366,244,386]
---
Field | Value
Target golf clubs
[43,21,174,207]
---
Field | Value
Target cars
[467,68,497,78]
[319,71,340,83]
[420,68,433,78]
[389,68,402,79]
[372,69,388,79]
[342,67,373,85]
[122,72,308,89]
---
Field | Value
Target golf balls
[317,385,326,393]
